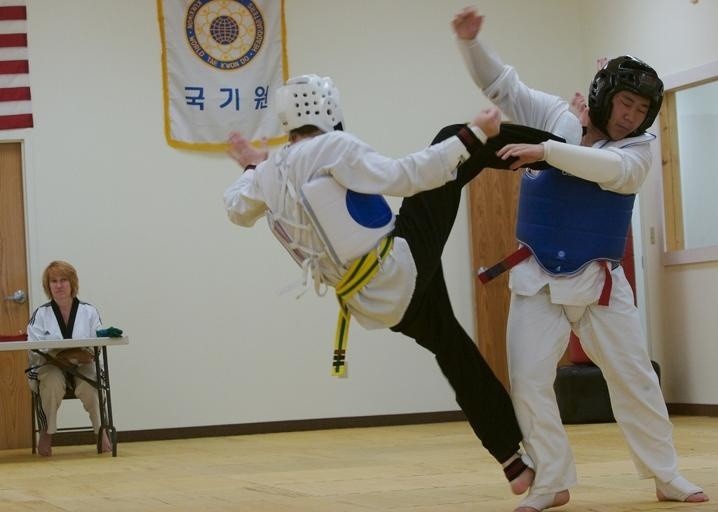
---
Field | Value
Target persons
[451,7,711,509]
[25,260,113,457]
[226,77,535,496]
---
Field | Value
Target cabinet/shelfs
[469,122,599,393]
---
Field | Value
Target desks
[0,336,129,457]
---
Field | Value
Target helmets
[588,55,665,137]
[275,74,346,132]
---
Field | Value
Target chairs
[32,368,106,454]
